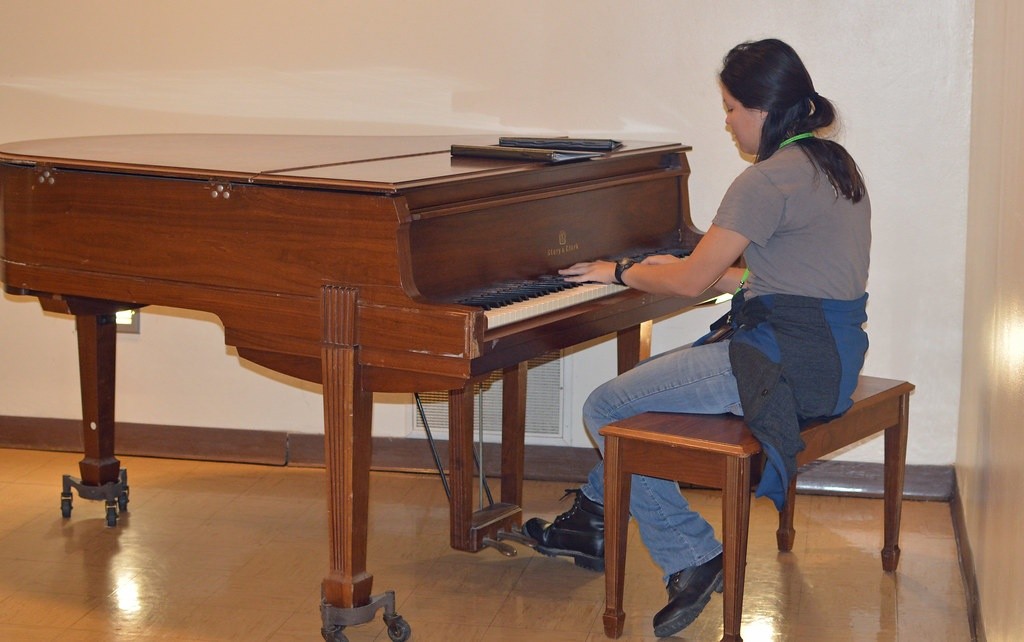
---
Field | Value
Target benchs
[595,375,916,642]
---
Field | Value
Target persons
[519,40,873,638]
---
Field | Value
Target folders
[498,134,623,152]
[450,142,606,164]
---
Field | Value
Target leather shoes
[521,488,605,574]
[652,552,723,637]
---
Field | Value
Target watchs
[615,258,634,286]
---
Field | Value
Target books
[450,137,624,162]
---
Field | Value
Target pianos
[2,131,747,642]
